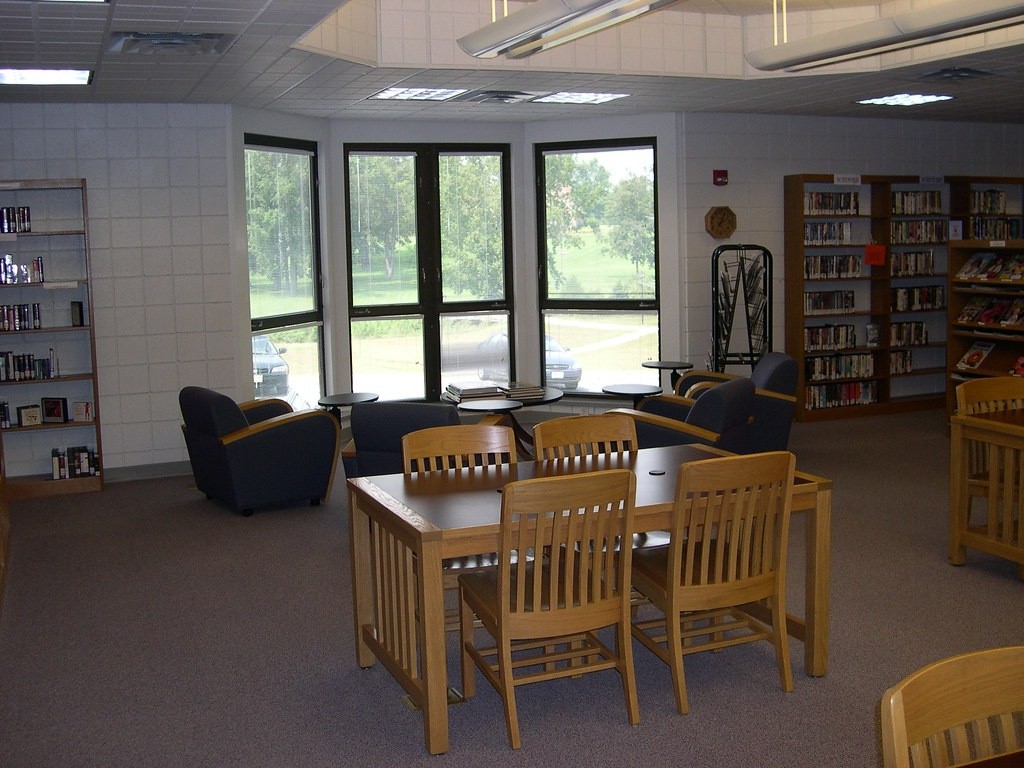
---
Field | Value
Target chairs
[872,646,1024,768]
[401,413,794,751]
[955,377,1024,535]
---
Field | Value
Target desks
[948,409,1024,567]
[439,375,565,461]
[345,443,835,756]
[318,387,380,413]
[601,357,694,401]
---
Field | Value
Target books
[705,190,1024,410]
[0,207,101,479]
[446,381,546,404]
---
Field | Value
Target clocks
[704,204,737,241]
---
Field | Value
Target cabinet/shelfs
[784,174,1024,425]
[0,177,107,499]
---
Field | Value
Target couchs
[177,348,798,518]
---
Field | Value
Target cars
[474,331,583,391]
[251,334,290,396]
